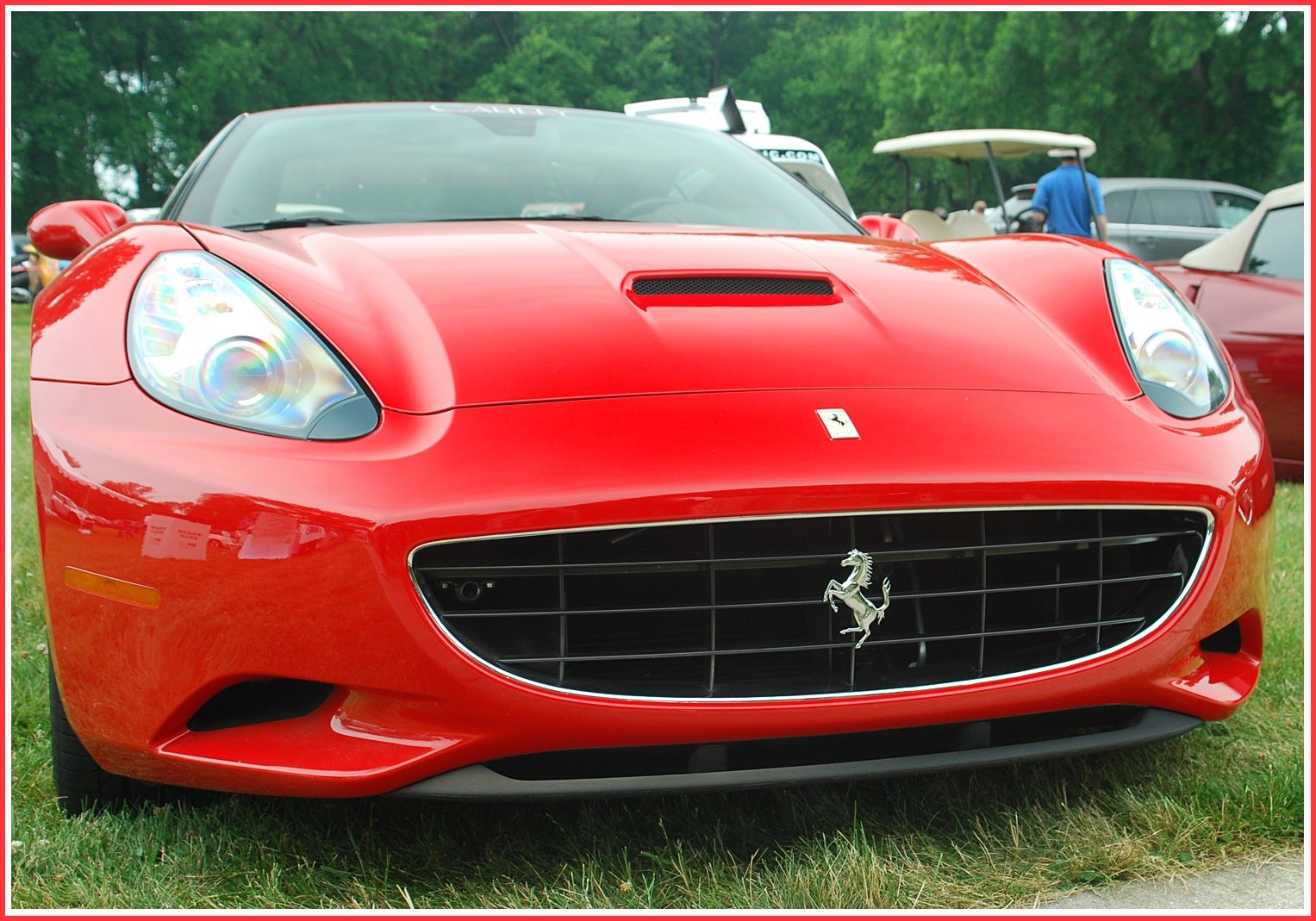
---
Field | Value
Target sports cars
[27,100,1275,829]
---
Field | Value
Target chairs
[558,139,762,217]
[902,209,953,242]
[280,137,549,221]
[946,210,997,240]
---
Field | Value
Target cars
[626,83,857,224]
[1144,179,1303,483]
[9,208,168,302]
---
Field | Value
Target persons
[18,251,45,307]
[1030,141,1108,242]
[971,199,1000,236]
[931,204,948,219]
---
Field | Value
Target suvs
[984,175,1264,265]
